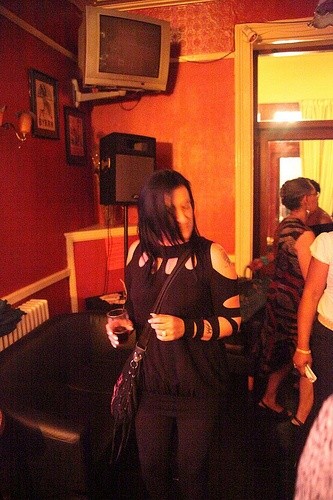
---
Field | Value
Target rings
[294,363,297,368]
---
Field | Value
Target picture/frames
[28,68,60,140]
[63,105,87,166]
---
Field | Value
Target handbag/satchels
[107,341,148,466]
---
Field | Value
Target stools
[223,337,254,391]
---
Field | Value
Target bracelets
[296,348,311,354]
[260,256,270,265]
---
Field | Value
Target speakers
[99,132,157,206]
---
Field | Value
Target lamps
[0,103,35,142]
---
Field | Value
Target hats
[282,178,316,201]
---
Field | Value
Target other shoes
[277,414,307,434]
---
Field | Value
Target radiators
[0,299,50,352]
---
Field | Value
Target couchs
[0,311,126,500]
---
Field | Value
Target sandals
[256,400,293,420]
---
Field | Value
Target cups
[107,308,130,345]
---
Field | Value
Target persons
[244,178,333,431]
[105,169,241,500]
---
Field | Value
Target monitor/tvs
[78,6,170,90]
